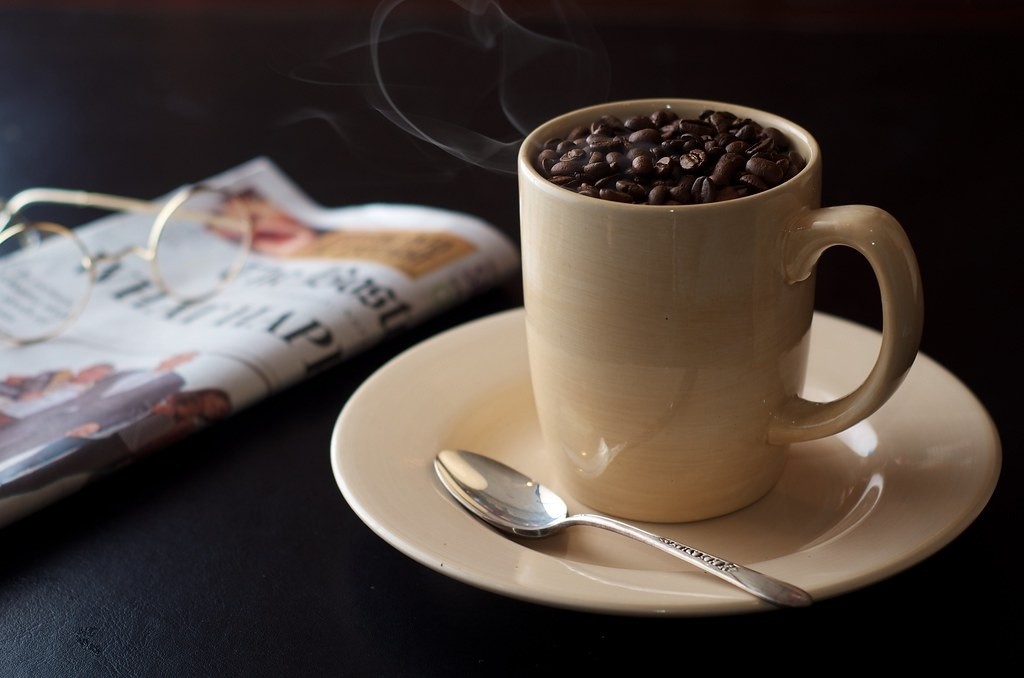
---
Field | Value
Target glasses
[1,179,260,348]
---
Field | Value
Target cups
[515,98,925,526]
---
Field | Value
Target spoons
[432,447,814,612]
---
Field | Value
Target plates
[328,302,1004,616]
